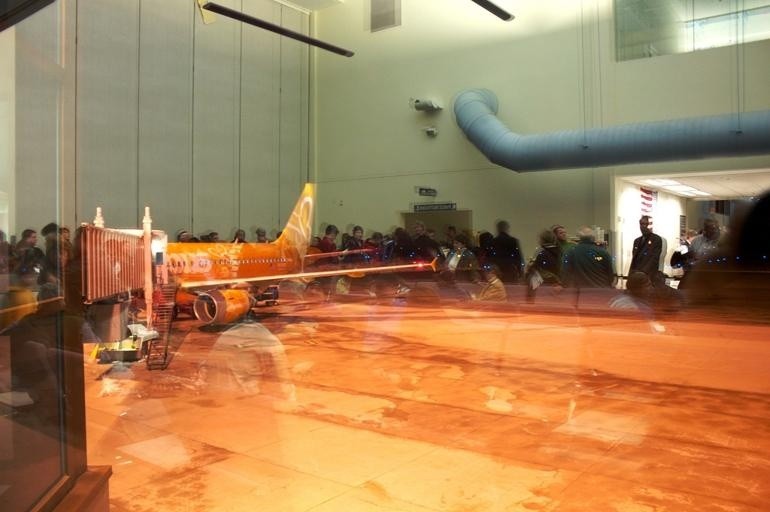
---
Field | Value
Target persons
[1,185,770,318]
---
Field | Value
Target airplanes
[153,180,438,327]
